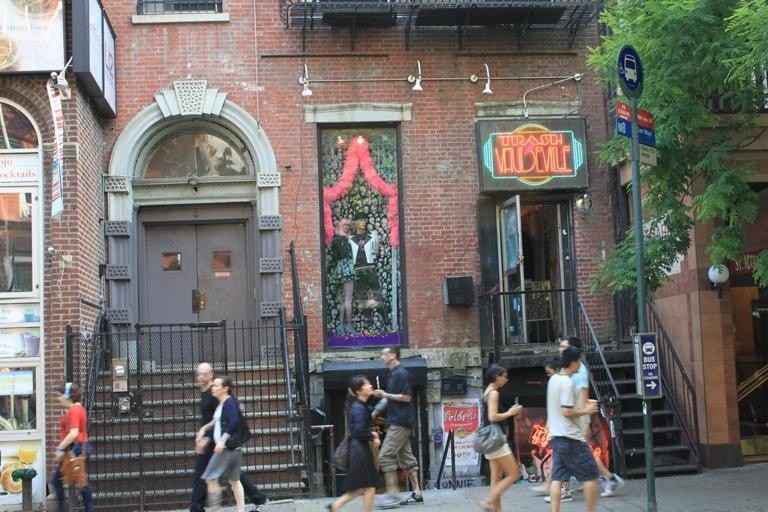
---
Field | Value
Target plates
[0,475,39,505]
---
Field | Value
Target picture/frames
[317,122,409,352]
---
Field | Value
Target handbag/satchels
[60,451,88,488]
[472,423,505,454]
[221,415,251,450]
[334,435,351,474]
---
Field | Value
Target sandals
[480,501,495,512]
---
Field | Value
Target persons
[479,365,523,512]
[371,346,423,509]
[195,376,245,512]
[546,346,601,512]
[190,363,270,512]
[528,335,624,503]
[327,375,381,512]
[332,212,396,336]
[52,382,93,512]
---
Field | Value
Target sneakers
[376,492,424,510]
[249,497,270,512]
[600,473,625,497]
[531,485,583,503]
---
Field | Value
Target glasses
[194,371,209,377]
[498,374,508,378]
[558,345,567,348]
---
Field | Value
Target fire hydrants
[13,468,37,511]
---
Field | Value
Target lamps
[298,62,583,97]
[707,263,730,299]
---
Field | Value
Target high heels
[325,503,332,512]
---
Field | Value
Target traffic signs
[638,331,663,399]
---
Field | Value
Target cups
[23,334,40,356]
[18,440,38,464]
[512,405,522,421]
[587,398,598,416]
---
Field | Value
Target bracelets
[56,448,66,451]
